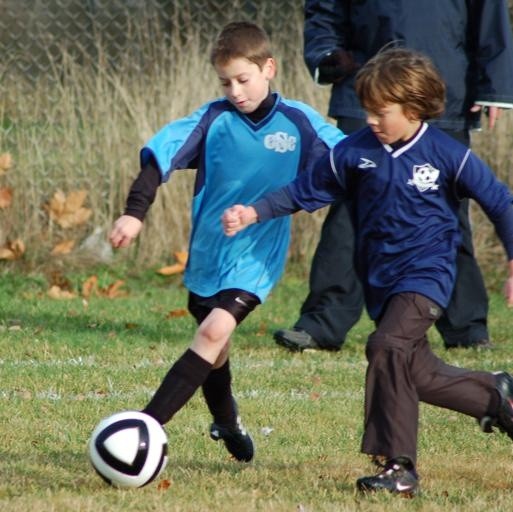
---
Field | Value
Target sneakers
[480,369,513,443]
[357,462,420,501]
[209,395,255,464]
[272,325,337,353]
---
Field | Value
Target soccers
[88,411,169,490]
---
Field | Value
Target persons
[272,0,511,353]
[217,41,510,497]
[107,20,351,464]
